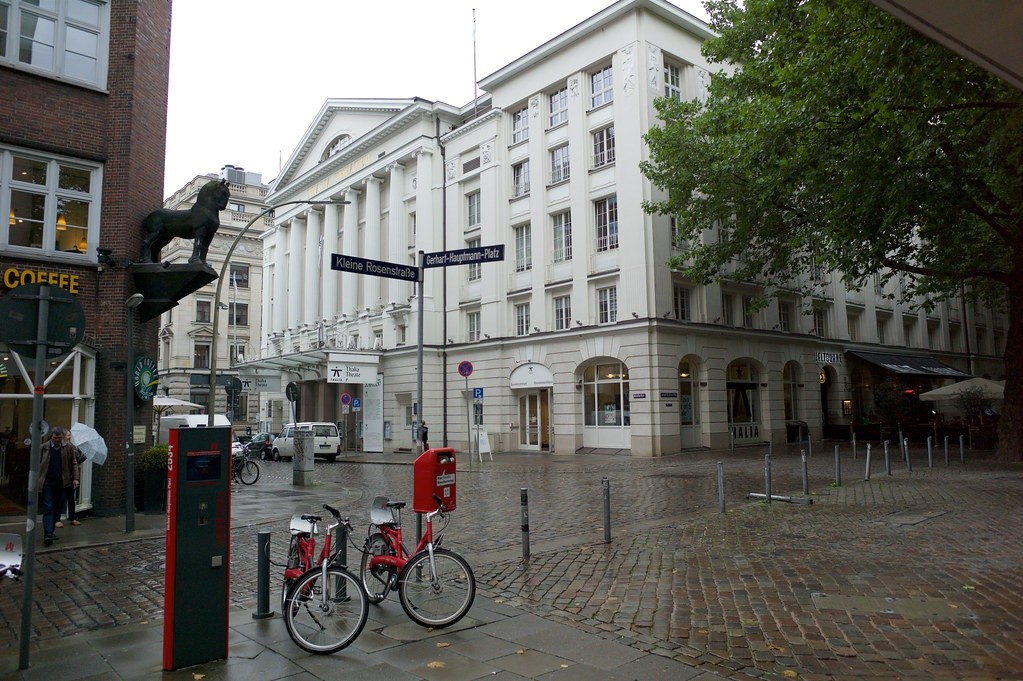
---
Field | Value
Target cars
[244,432,280,461]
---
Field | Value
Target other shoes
[71,521,83,525]
[43,536,54,544]
[54,533,60,541]
[56,522,64,528]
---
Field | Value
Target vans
[271,421,342,463]
[156,413,248,464]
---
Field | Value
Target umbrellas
[919,378,1007,401]
[153,398,205,445]
[69,422,109,466]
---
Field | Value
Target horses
[136,178,231,263]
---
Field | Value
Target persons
[55,430,88,529]
[38,427,79,545]
[418,423,430,451]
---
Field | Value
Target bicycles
[360,492,477,629]
[232,445,260,486]
[282,504,370,656]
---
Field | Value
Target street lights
[207,200,352,426]
[125,292,144,534]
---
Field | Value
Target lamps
[680,371,689,377]
[605,366,625,378]
[77,229,87,252]
[8,208,15,224]
[21,168,27,175]
[57,215,67,230]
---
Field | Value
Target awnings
[845,350,973,375]
